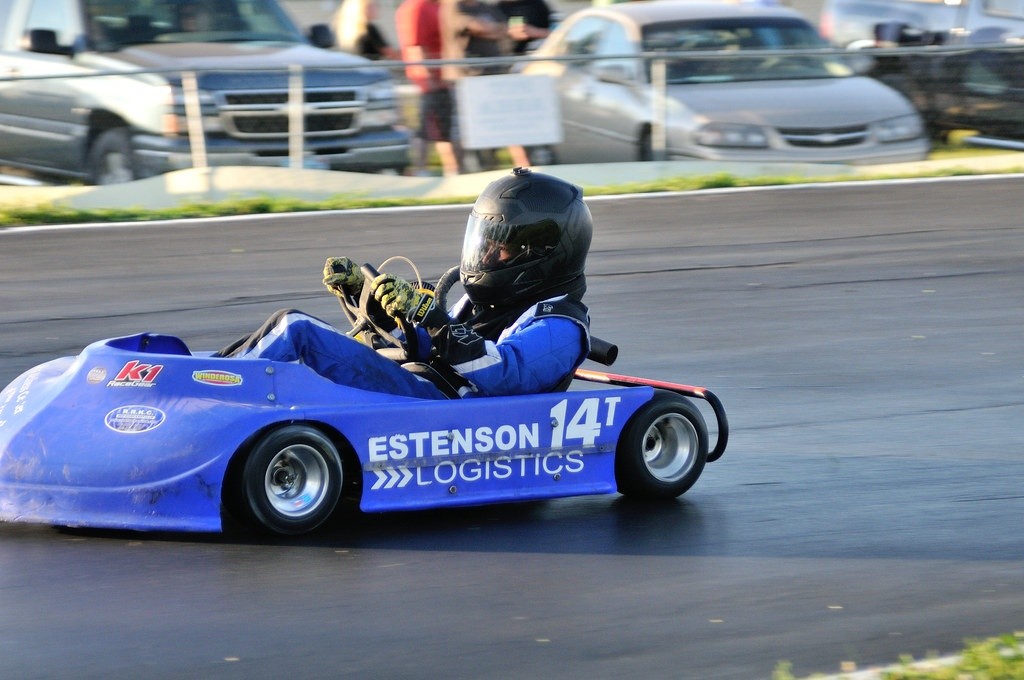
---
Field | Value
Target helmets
[460,166,594,304]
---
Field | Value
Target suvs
[0,0,418,186]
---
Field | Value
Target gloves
[322,256,364,298]
[370,273,451,330]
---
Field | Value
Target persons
[209,167,593,400]
[303,0,552,175]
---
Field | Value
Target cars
[818,0,1024,142]
[512,1,932,165]
